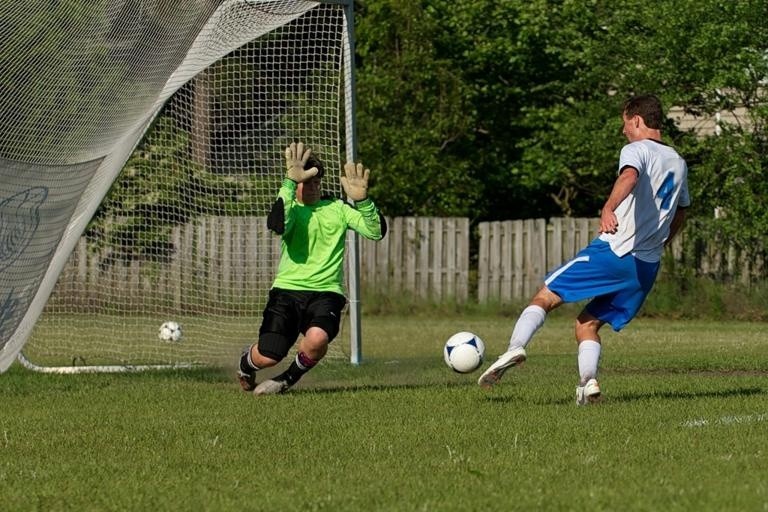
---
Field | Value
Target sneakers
[253,372,296,396]
[478,347,526,386]
[576,379,601,406]
[238,348,256,391]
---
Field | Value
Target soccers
[444,332,486,374]
[158,321,183,343]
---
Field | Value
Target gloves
[340,161,370,201]
[285,143,318,183]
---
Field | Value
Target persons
[237,142,387,396]
[478,95,691,405]
